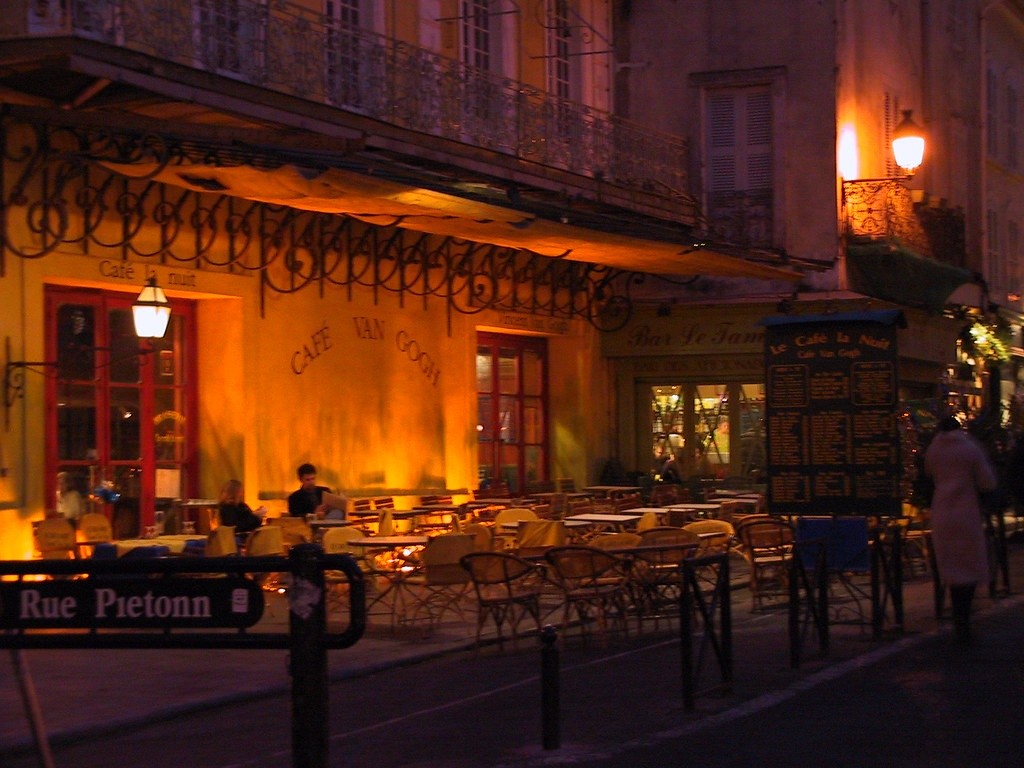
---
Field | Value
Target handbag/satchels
[909,458,935,507]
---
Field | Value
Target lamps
[840,109,927,213]
[0,269,173,434]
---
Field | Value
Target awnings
[844,239,992,321]
[98,161,803,281]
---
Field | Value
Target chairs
[32,476,930,656]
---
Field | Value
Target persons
[55,471,86,524]
[923,414,998,644]
[217,478,266,543]
[287,463,332,517]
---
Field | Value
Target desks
[707,497,758,510]
[697,489,755,496]
[664,503,721,520]
[530,492,592,505]
[347,535,434,636]
[621,506,696,526]
[581,485,645,499]
[349,509,429,537]
[468,498,537,510]
[158,534,209,557]
[413,504,461,534]
[564,512,642,532]
[309,518,352,535]
[75,538,187,577]
[501,520,595,545]
